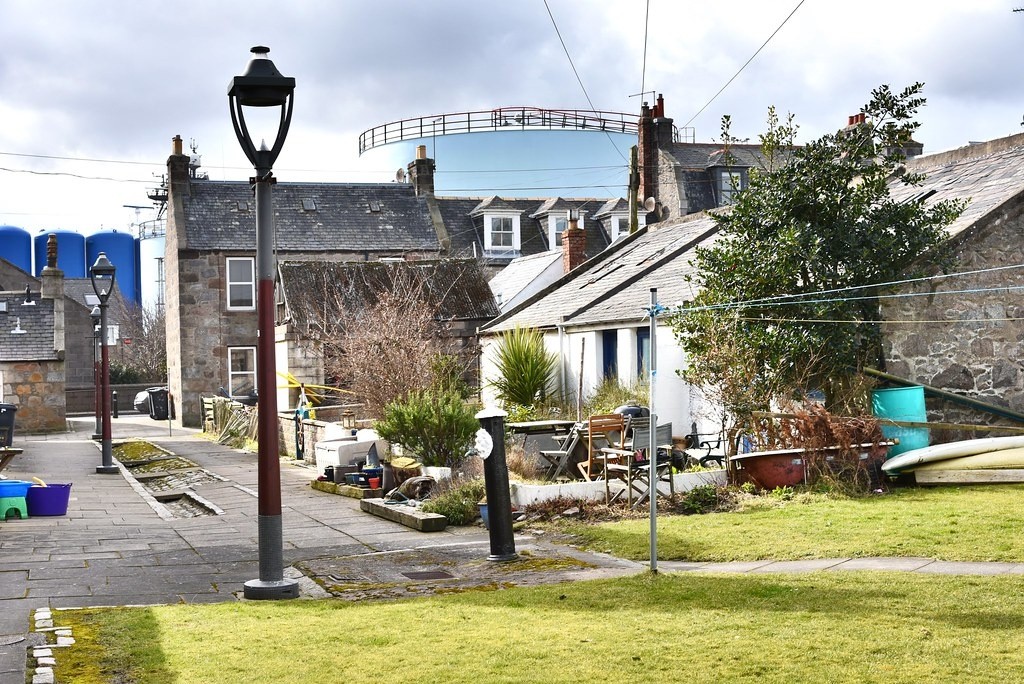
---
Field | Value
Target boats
[727,439,897,492]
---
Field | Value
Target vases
[345,472,383,488]
[477,503,490,531]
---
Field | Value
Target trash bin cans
[146,387,168,420]
[0,402,17,448]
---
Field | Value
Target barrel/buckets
[325,464,380,489]
[869,385,929,460]
[0,479,73,516]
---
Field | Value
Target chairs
[601,422,674,511]
[576,412,658,482]
[540,422,586,482]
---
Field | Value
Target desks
[506,419,579,450]
[0,447,23,472]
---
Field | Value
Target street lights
[88,304,103,439]
[88,251,121,474]
[227,45,300,600]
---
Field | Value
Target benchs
[684,428,742,467]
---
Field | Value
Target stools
[0,497,28,520]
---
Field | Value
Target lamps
[340,408,355,430]
